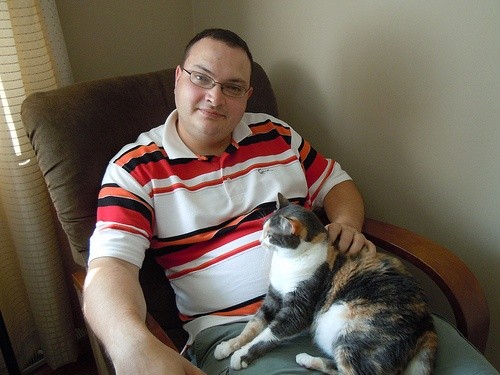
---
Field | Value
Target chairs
[19,59,491,375]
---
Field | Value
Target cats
[213,191,441,375]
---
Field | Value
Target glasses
[181,67,250,98]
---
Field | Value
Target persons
[81,27,500,375]
